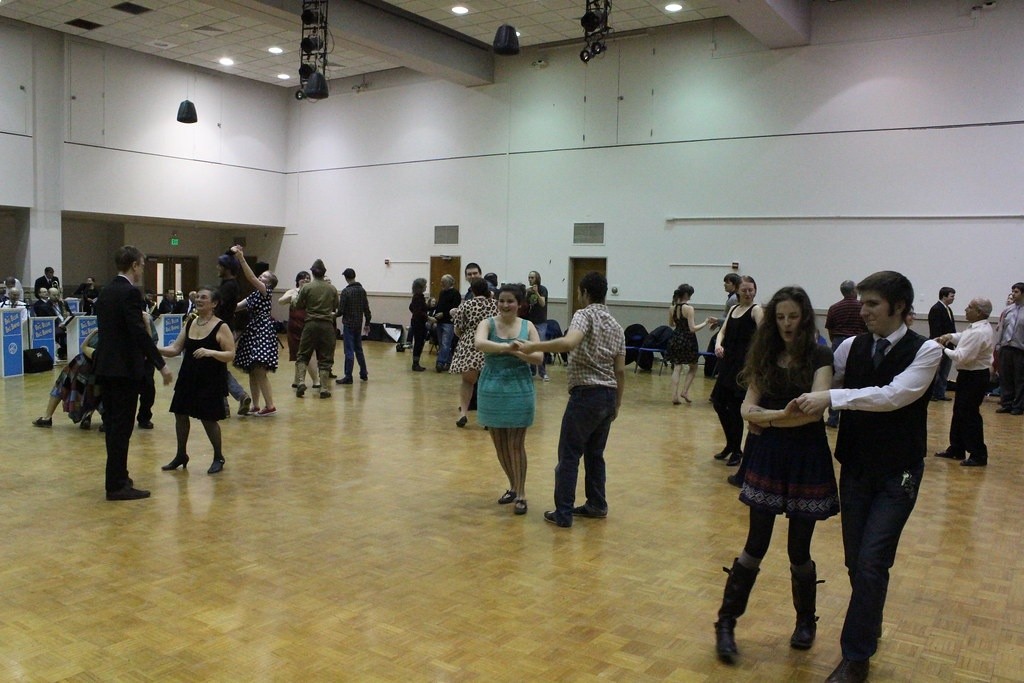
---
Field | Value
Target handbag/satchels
[24,347,54,373]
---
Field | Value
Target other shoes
[543,511,571,527]
[714,448,732,459]
[412,364,426,371]
[826,658,869,683]
[935,451,953,458]
[514,500,528,514]
[726,453,742,465]
[996,407,1023,415]
[291,372,353,387]
[498,491,517,503]
[572,506,608,517]
[961,459,987,465]
[360,376,368,380]
[296,385,306,397]
[138,422,153,428]
[81,416,106,432]
[226,394,251,418]
[32,417,52,426]
[320,392,331,398]
[931,396,952,401]
[106,478,150,499]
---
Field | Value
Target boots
[790,561,825,647]
[714,557,761,656]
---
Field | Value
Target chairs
[544,328,721,380]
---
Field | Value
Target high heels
[456,416,468,428]
[208,458,225,473]
[162,456,189,470]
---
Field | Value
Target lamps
[579,0,612,62]
[175,0,198,124]
[304,0,329,100]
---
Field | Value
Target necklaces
[196,313,214,326]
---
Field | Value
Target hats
[218,254,241,272]
[310,260,326,273]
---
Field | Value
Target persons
[933,296,995,467]
[474,285,544,515]
[711,275,764,465]
[464,263,500,300]
[525,271,549,382]
[826,281,871,428]
[436,274,461,372]
[404,297,436,349]
[449,278,500,431]
[714,287,841,661]
[669,284,709,403]
[906,303,915,330]
[996,283,1024,415]
[0,244,338,499]
[518,271,625,529]
[709,273,739,332]
[331,268,371,385]
[409,278,427,372]
[928,287,956,401]
[791,271,943,683]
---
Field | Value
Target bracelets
[769,420,772,427]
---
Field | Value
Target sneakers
[246,406,277,416]
